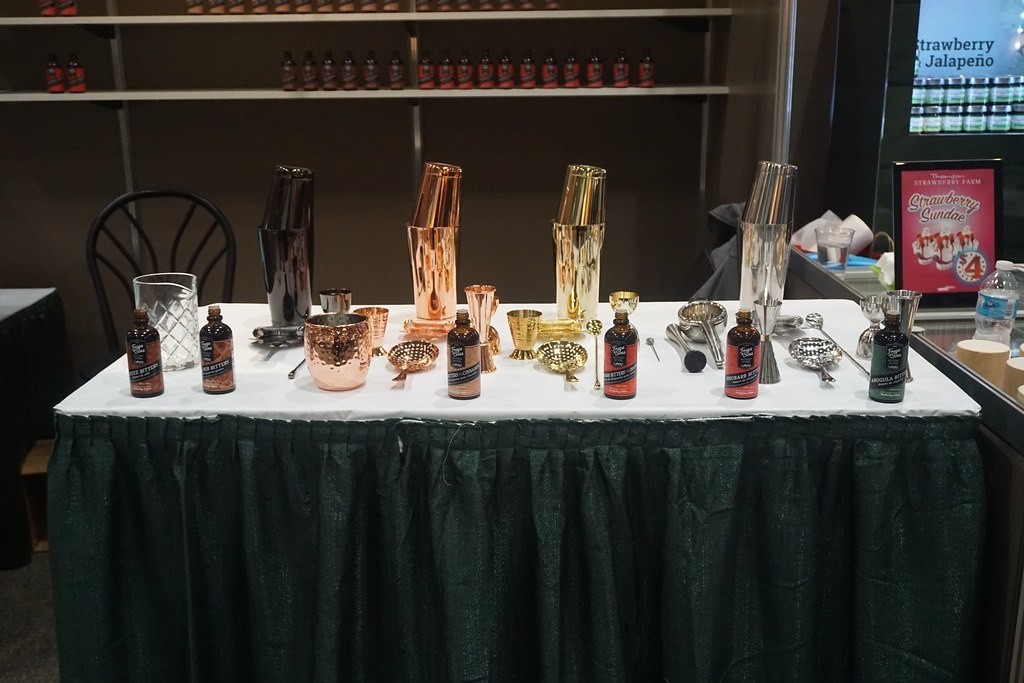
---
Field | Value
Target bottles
[278,49,655,92]
[972,260,1024,348]
[42,50,85,93]
[724,310,760,400]
[199,306,236,395]
[909,77,1024,133]
[125,307,163,398]
[40,1,77,15]
[869,311,906,403]
[605,310,638,399]
[446,310,480,400]
[188,0,559,15]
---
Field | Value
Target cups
[506,310,542,359]
[134,274,197,371]
[886,289,923,383]
[741,160,798,224]
[258,224,312,327]
[412,162,461,227]
[555,165,604,225]
[815,226,855,271]
[351,306,388,357]
[550,218,604,331]
[740,218,791,342]
[304,313,373,390]
[855,296,899,360]
[957,337,1024,408]
[608,291,639,316]
[405,220,458,319]
[318,287,350,313]
[262,164,313,229]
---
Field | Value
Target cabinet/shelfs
[0,0,734,271]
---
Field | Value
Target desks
[44,297,989,683]
[0,287,75,571]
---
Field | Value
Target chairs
[87,188,238,361]
